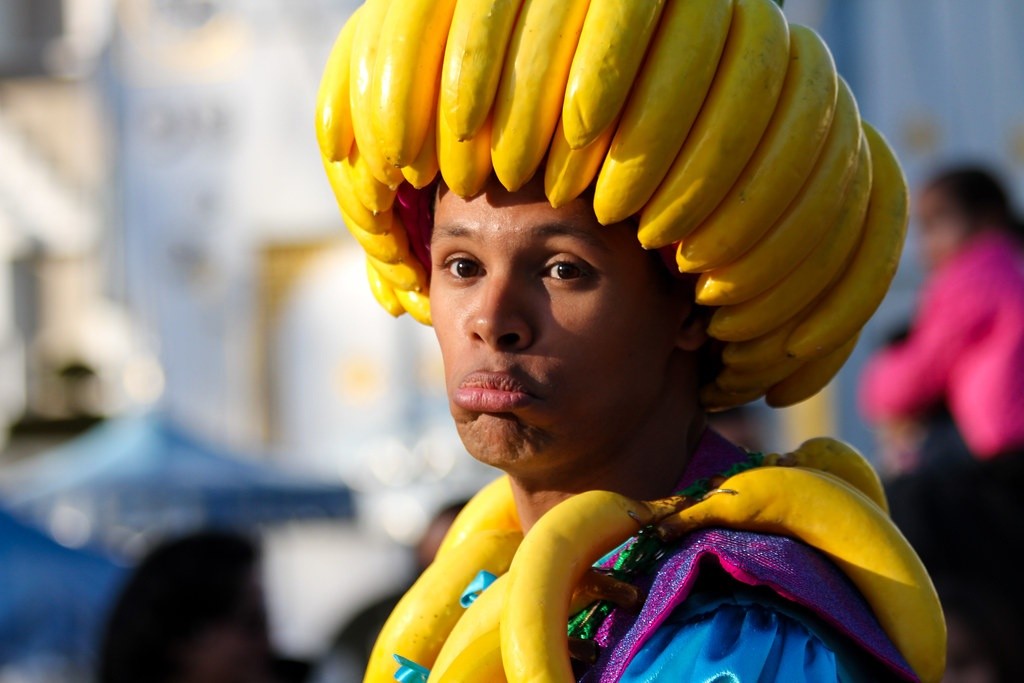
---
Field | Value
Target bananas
[357,435,949,683]
[310,0,909,412]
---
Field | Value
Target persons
[858,166,1024,683]
[316,0,949,683]
[6,361,104,452]
[92,498,471,683]
